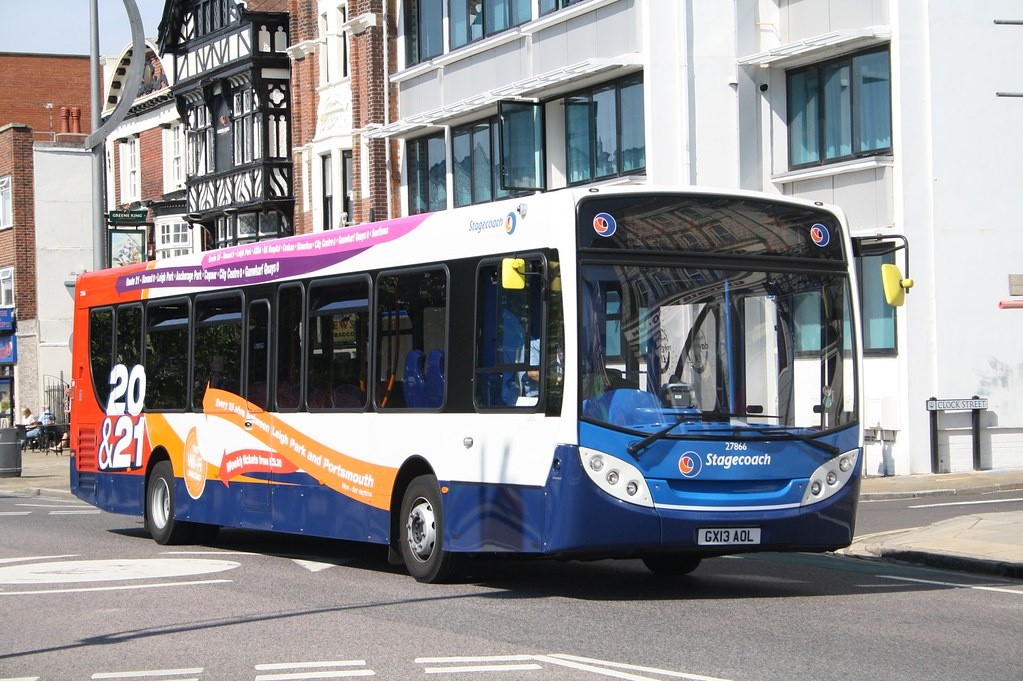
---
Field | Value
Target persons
[12,407,35,450]
[49,388,71,452]
[21,403,56,451]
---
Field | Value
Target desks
[45,424,69,452]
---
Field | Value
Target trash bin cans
[0,428,23,478]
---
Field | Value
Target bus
[68,183,914,585]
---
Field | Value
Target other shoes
[50,447,62,453]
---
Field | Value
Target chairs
[211,349,444,408]
[16,422,62,456]
[605,369,639,388]
